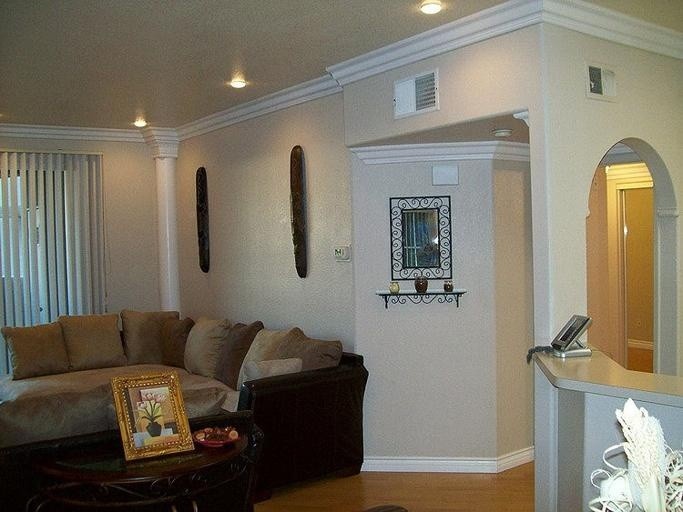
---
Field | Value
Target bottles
[389,276,454,295]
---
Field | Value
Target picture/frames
[110,369,195,461]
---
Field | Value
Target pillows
[236,328,292,391]
[182,317,237,381]
[223,319,266,391]
[263,324,344,376]
[242,356,303,386]
[166,314,196,370]
[1,318,72,381]
[58,311,129,374]
[120,310,181,367]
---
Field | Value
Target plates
[192,428,239,448]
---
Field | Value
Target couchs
[0,320,366,511]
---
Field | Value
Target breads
[203,427,213,434]
[194,432,206,440]
[229,430,239,440]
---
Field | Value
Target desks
[24,409,264,511]
